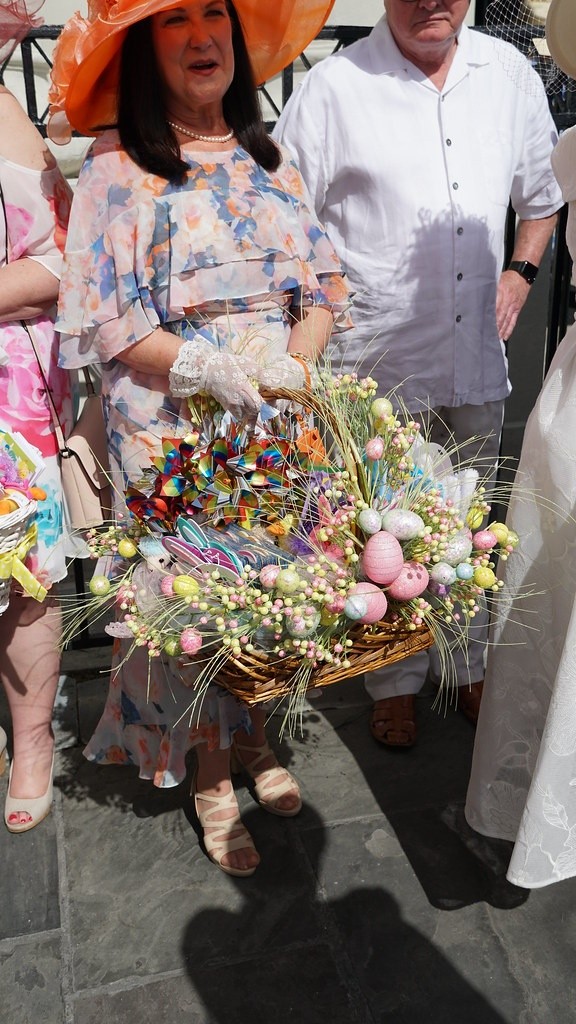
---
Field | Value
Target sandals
[369,695,417,751]
[4,744,56,833]
[453,679,484,727]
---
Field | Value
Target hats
[45,0,335,138]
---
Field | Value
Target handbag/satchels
[59,395,118,531]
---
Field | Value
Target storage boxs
[1,489,38,617]
[175,386,438,704]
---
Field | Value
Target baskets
[175,386,437,707]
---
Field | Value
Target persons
[1,1,575,890]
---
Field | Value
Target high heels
[188,769,260,877]
[232,731,303,817]
